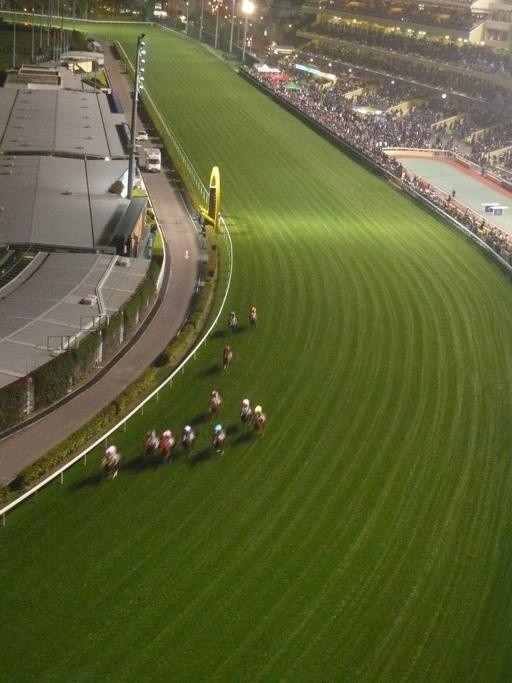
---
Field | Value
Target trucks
[143,147,162,174]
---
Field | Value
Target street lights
[126,30,148,200]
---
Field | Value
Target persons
[230,311,236,321]
[237,0,512,174]
[467,165,511,182]
[391,160,438,204]
[251,305,257,318]
[102,389,265,472]
[224,343,232,356]
[434,188,510,264]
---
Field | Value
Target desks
[481,203,508,215]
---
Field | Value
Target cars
[136,130,149,142]
